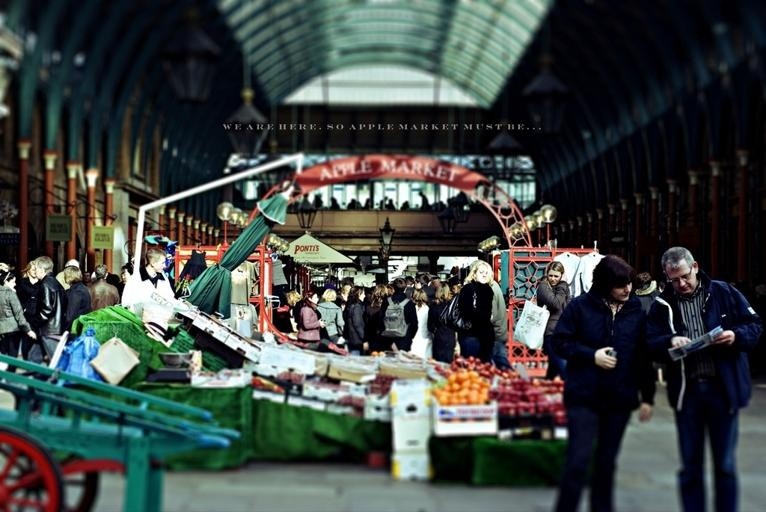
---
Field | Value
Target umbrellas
[174,187,294,319]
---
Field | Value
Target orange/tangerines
[433,369,490,406]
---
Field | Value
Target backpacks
[382,297,410,338]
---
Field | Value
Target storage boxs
[192,313,496,478]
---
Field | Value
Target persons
[273,188,533,215]
[548,253,661,512]
[0,243,711,374]
[629,245,764,510]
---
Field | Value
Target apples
[435,353,566,425]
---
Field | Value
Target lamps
[438,0,472,234]
[225,0,319,228]
[379,216,396,260]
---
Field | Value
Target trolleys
[0,348,244,512]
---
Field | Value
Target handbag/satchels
[441,292,477,329]
[513,300,550,350]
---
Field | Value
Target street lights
[379,216,396,283]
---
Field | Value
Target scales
[146,367,190,382]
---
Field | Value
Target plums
[376,376,399,395]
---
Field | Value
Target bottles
[70,329,104,384]
